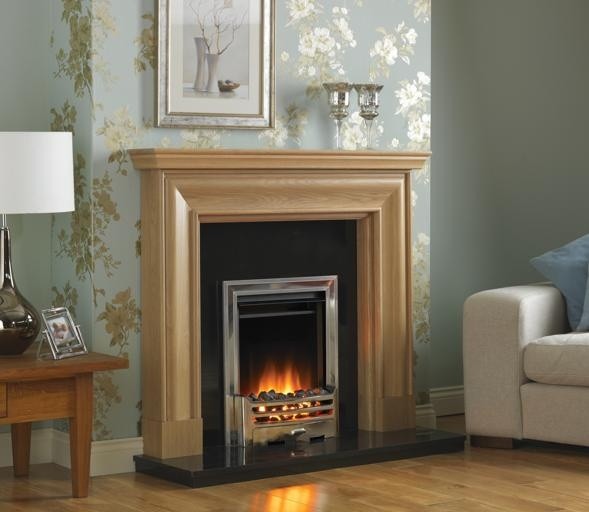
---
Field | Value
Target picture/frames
[39,305,89,360]
[154,0,279,133]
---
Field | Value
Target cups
[354,83,385,151]
[323,82,354,151]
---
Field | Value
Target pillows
[528,231,588,331]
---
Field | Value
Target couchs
[459,281,589,450]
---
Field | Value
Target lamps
[0,130,74,216]
